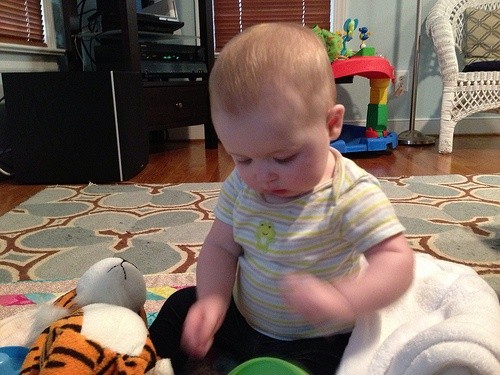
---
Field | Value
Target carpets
[0,172,500,318]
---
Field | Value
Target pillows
[464,7,500,65]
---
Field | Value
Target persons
[149,22,415,375]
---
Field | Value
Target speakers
[3,70,148,186]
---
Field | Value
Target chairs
[425,0,500,153]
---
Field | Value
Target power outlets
[394,70,408,93]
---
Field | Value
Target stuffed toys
[19,256,174,375]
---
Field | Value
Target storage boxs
[1,72,148,185]
